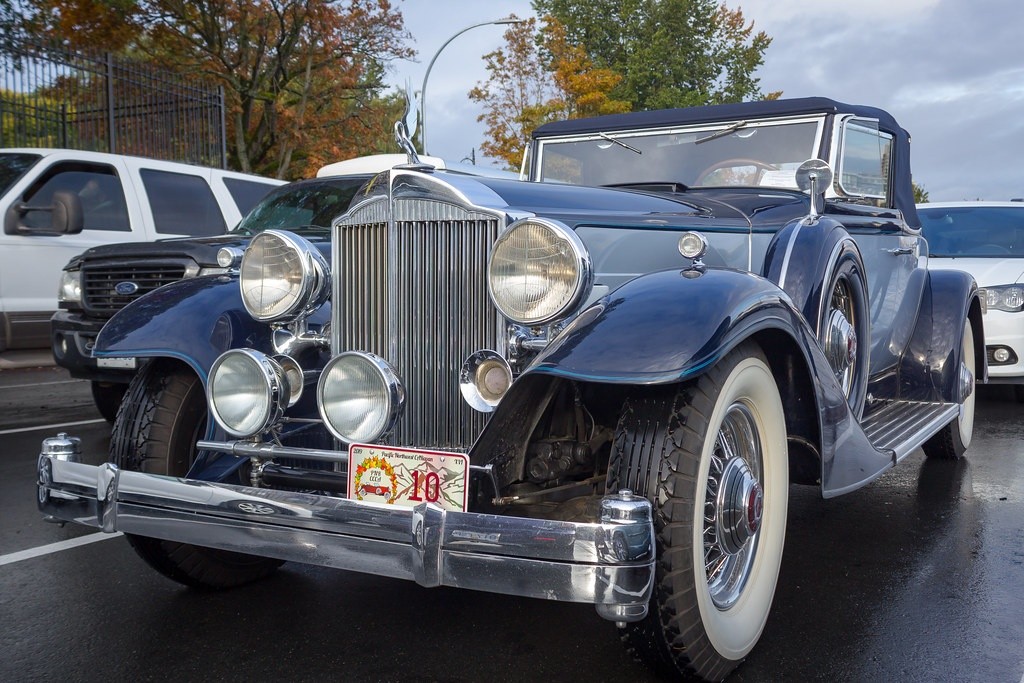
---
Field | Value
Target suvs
[0,148,292,352]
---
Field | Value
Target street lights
[420,18,524,155]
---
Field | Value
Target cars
[913,201,1024,385]
[35,93,989,682]
